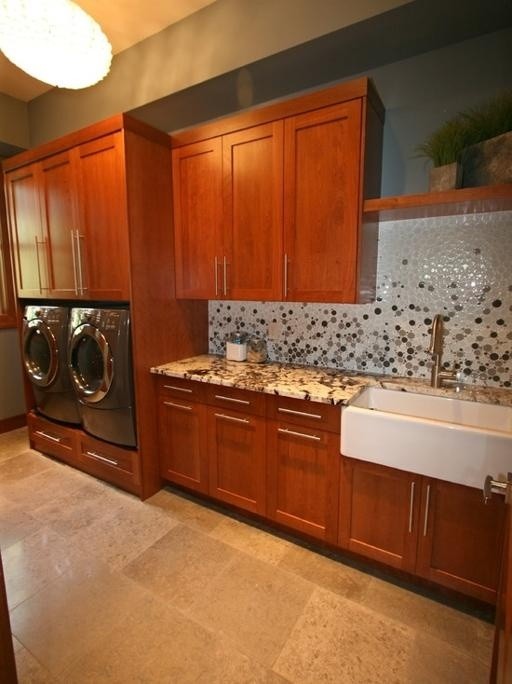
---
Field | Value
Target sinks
[338,388,512,493]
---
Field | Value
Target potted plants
[409,121,467,192]
[464,88,512,188]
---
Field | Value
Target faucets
[427,313,457,389]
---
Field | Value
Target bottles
[246,335,268,363]
[225,329,248,361]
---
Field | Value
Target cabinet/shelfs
[339,452,507,608]
[282,96,364,304]
[170,118,283,303]
[210,383,268,518]
[157,375,210,496]
[6,160,52,298]
[41,128,130,301]
[267,394,337,547]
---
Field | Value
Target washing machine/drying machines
[67,306,136,450]
[20,305,80,425]
